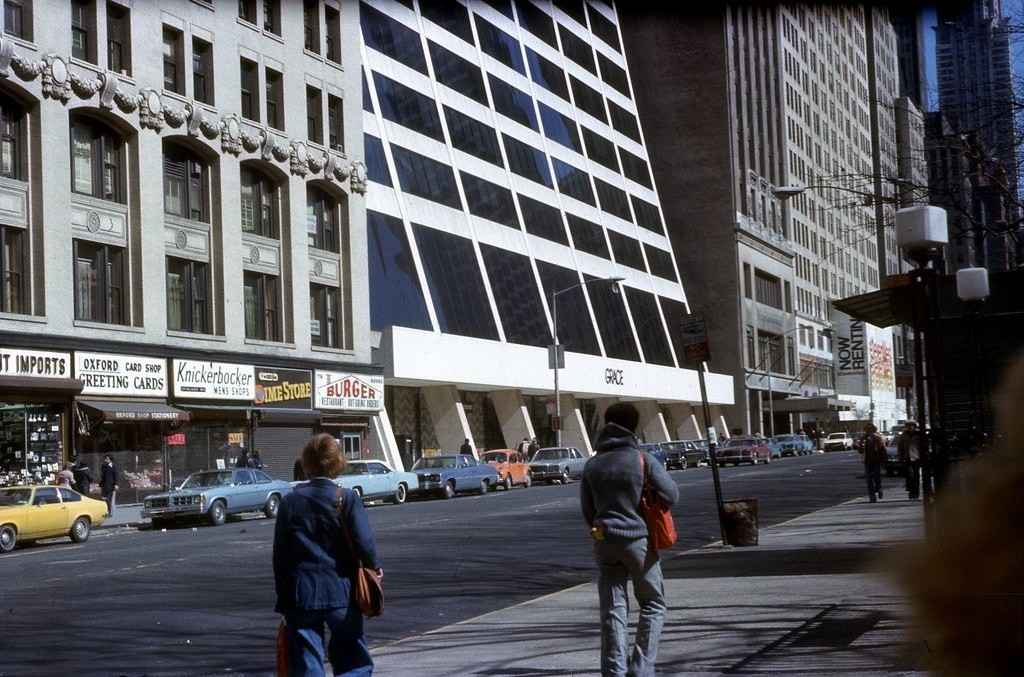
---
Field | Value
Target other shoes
[908,490,920,501]
[878,489,883,499]
[869,497,876,503]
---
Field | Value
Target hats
[904,420,916,426]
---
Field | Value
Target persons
[719,433,726,443]
[271,432,383,677]
[528,437,540,462]
[251,450,268,470]
[580,402,679,677]
[98,455,119,518]
[460,439,474,456]
[236,448,256,468]
[518,437,532,463]
[897,420,923,501]
[858,423,889,503]
[799,427,825,450]
[58,456,94,497]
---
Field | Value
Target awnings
[78,400,191,420]
[831,287,899,329]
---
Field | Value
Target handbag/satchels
[641,493,678,549]
[354,562,386,618]
[860,452,865,462]
[277,621,289,677]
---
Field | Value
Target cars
[291,459,420,507]
[656,440,708,470]
[636,443,669,471]
[717,436,771,466]
[528,447,588,484]
[762,438,783,459]
[693,439,720,466]
[479,448,532,490]
[0,485,110,552]
[410,454,498,498]
[823,432,854,452]
[885,433,904,477]
[773,434,804,457]
[139,467,293,527]
[798,435,815,455]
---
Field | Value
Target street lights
[551,275,627,454]
[771,186,932,535]
